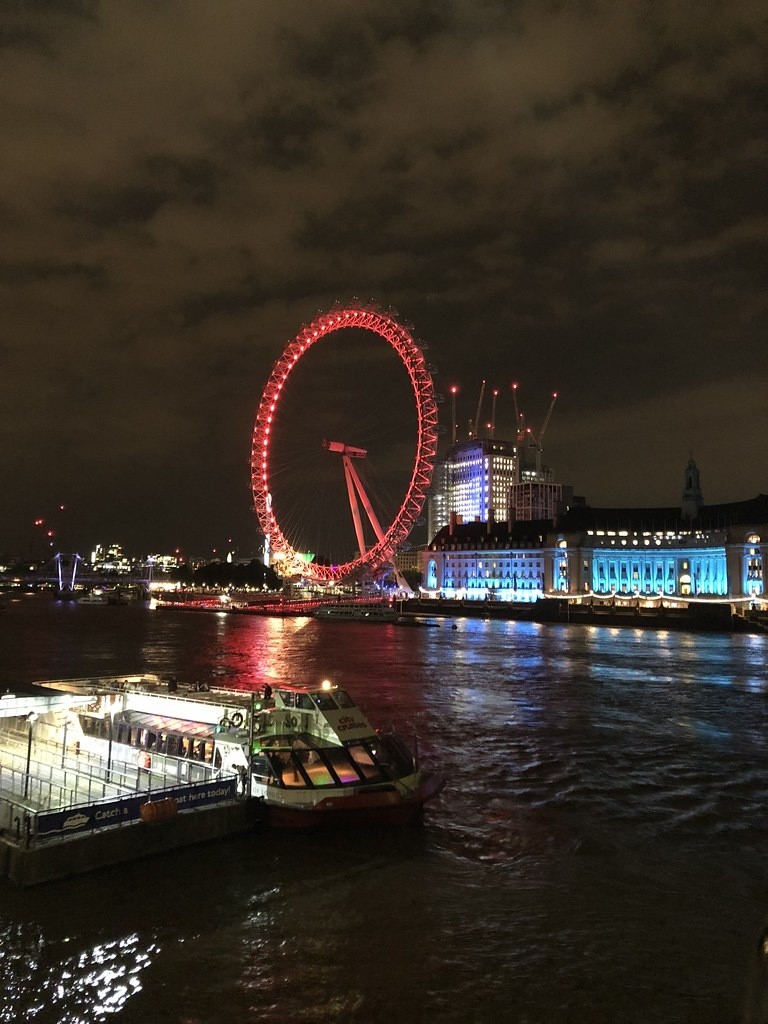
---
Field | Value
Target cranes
[466,379,485,440]
[451,387,457,443]
[527,392,559,452]
[487,389,498,438]
[512,384,524,445]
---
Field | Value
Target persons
[231,764,247,796]
[293,696,303,709]
[145,730,168,753]
[284,692,291,707]
[105,680,118,691]
[263,683,272,699]
[267,751,284,786]
[122,680,144,692]
[168,675,178,693]
[287,750,299,782]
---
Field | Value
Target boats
[32,673,446,827]
[77,597,108,605]
[314,600,399,622]
